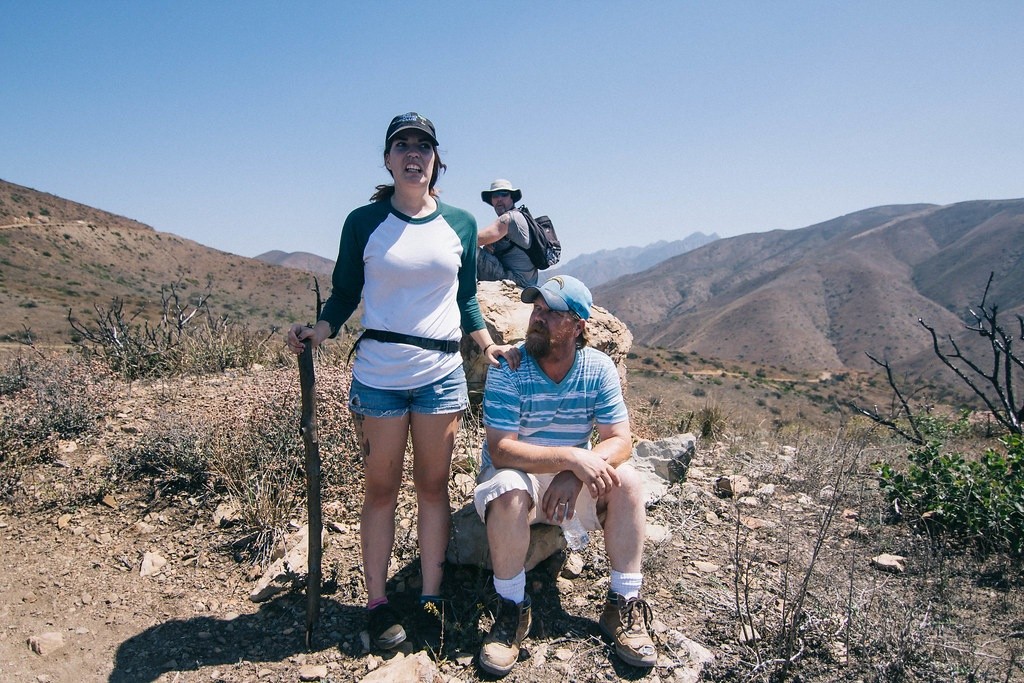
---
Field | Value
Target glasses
[491,191,509,198]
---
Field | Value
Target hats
[521,274,592,320]
[386,111,439,146]
[481,178,522,206]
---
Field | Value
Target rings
[560,502,565,506]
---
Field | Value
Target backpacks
[503,205,562,270]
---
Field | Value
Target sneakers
[368,601,407,650]
[598,587,656,666]
[479,592,532,675]
[414,603,477,644]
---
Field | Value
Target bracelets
[484,343,495,355]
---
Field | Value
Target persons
[476,179,538,289]
[474,275,658,676]
[286,112,522,650]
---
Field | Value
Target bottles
[554,503,591,550]
